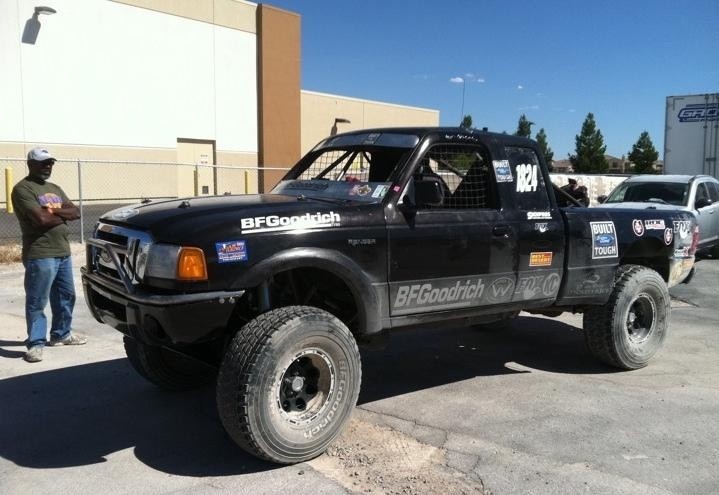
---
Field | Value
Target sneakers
[25,346,43,362]
[50,332,88,346]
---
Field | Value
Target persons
[11,146,89,364]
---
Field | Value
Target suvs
[76,125,700,471]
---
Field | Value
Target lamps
[334,118,351,124]
[31,5,58,18]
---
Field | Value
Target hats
[27,146,57,163]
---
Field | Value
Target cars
[596,168,719,263]
